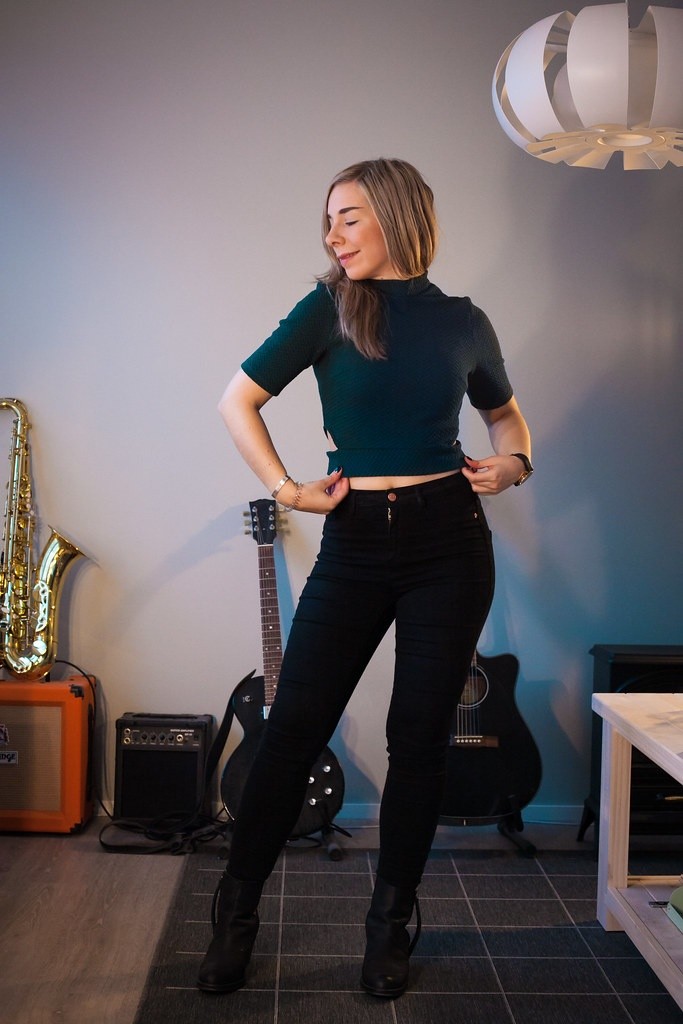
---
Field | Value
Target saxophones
[1,392,103,682]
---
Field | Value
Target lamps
[491,0,683,170]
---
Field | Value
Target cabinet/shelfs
[592,692,683,1015]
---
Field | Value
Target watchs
[510,453,534,487]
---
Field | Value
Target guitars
[431,452,544,829]
[218,498,345,844]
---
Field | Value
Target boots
[363,878,421,996]
[197,869,267,992]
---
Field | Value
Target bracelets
[286,482,302,512]
[270,475,291,498]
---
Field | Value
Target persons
[198,155,532,996]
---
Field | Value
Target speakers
[0,673,96,834]
[112,711,216,838]
